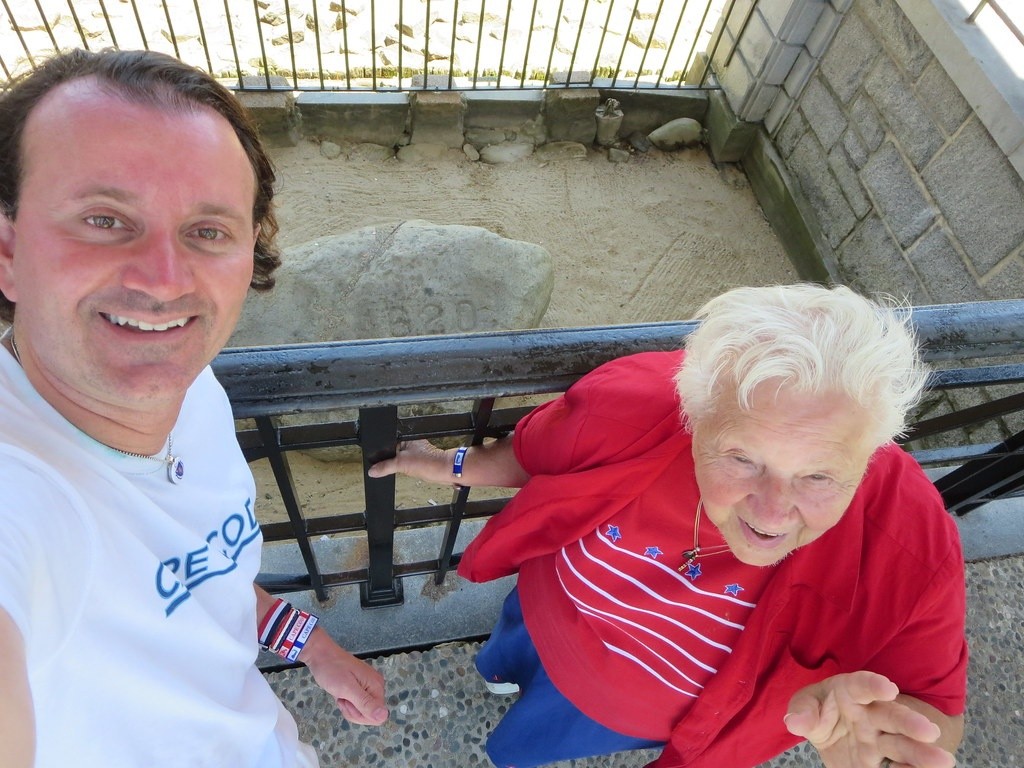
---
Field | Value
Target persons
[0,48,388,767]
[367,284,968,768]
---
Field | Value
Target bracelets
[451,448,469,492]
[257,597,319,661]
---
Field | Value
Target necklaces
[678,498,731,574]
[9,334,186,486]
